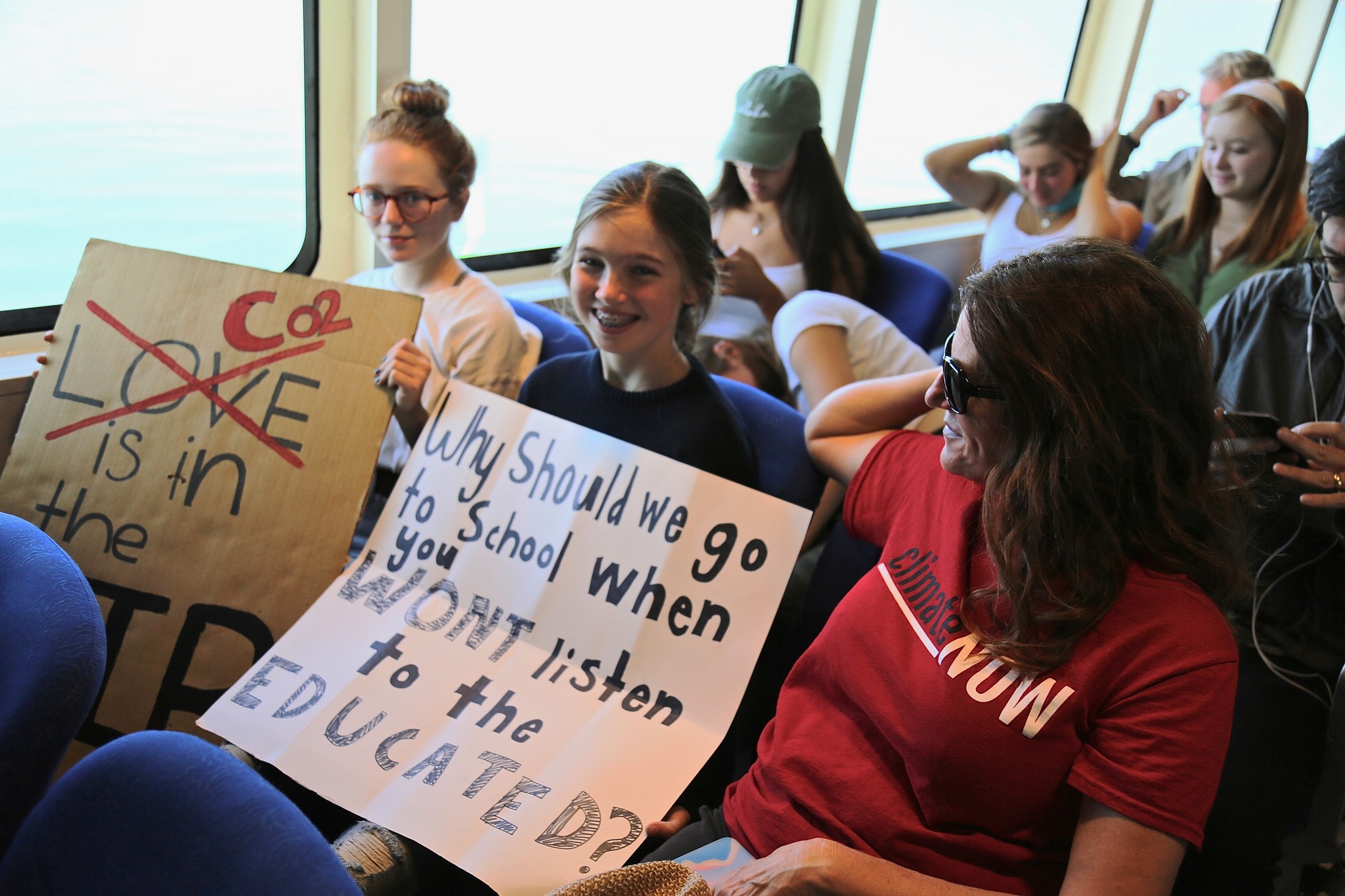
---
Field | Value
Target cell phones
[1222,411,1310,470]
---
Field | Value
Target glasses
[347,186,465,221]
[943,330,1015,414]
[1302,222,1345,284]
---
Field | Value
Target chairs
[0,510,363,896]
[510,250,955,503]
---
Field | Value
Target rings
[1333,472,1341,490]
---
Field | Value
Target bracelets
[1126,133,1140,148]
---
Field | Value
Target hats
[716,63,822,168]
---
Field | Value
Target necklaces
[1210,225,1230,257]
[737,207,776,236]
[1031,207,1067,230]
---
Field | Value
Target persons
[222,163,760,896]
[696,65,876,340]
[1201,137,1345,896]
[629,243,1235,896]
[925,104,1141,272]
[1140,79,1321,317]
[688,293,949,555]
[31,81,520,571]
[1108,49,1278,230]
